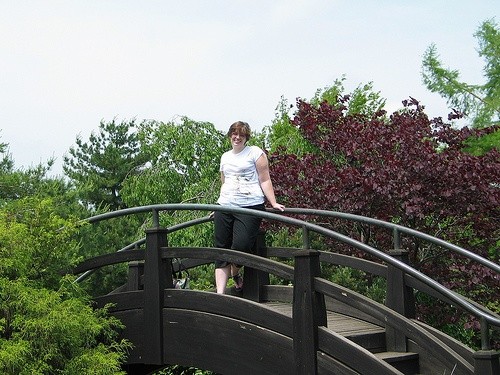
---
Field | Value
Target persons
[214,119,286,296]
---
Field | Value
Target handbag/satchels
[171,257,190,289]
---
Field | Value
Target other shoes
[232,273,243,289]
